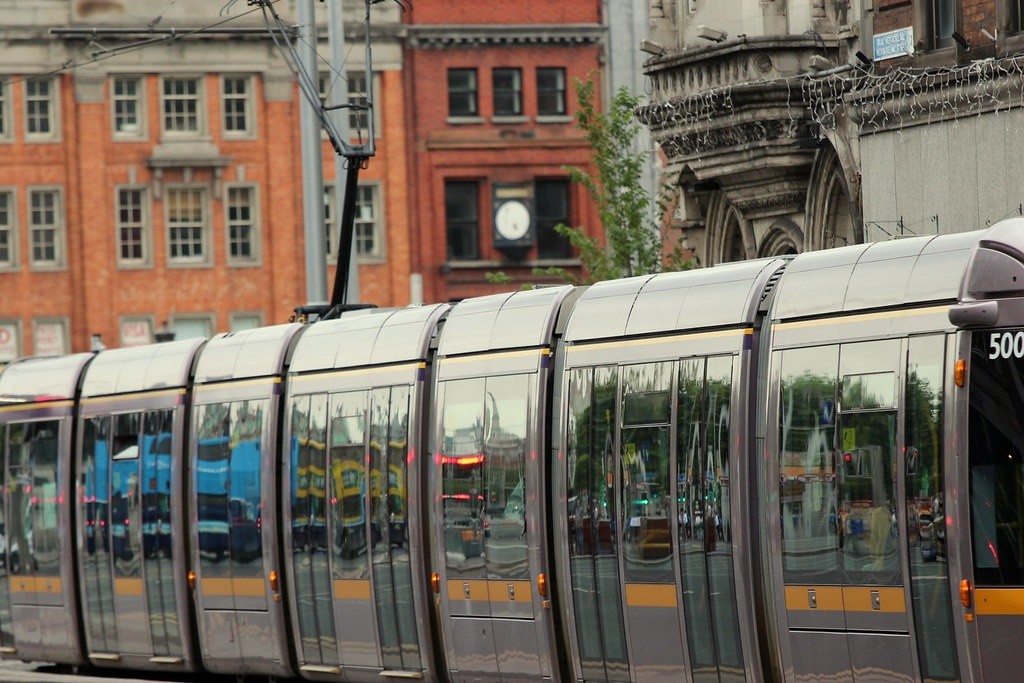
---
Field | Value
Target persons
[574,496,730,559]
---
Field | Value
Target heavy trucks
[86,432,299,563]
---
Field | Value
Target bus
[1,217,1024,683]
